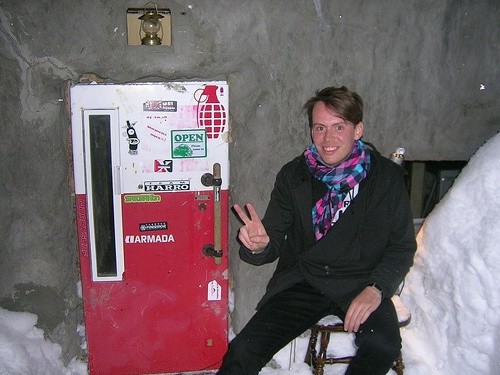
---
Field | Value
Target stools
[304,295,411,375]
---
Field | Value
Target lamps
[137,1,165,45]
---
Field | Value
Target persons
[215,87,418,375]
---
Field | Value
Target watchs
[365,282,385,302]
